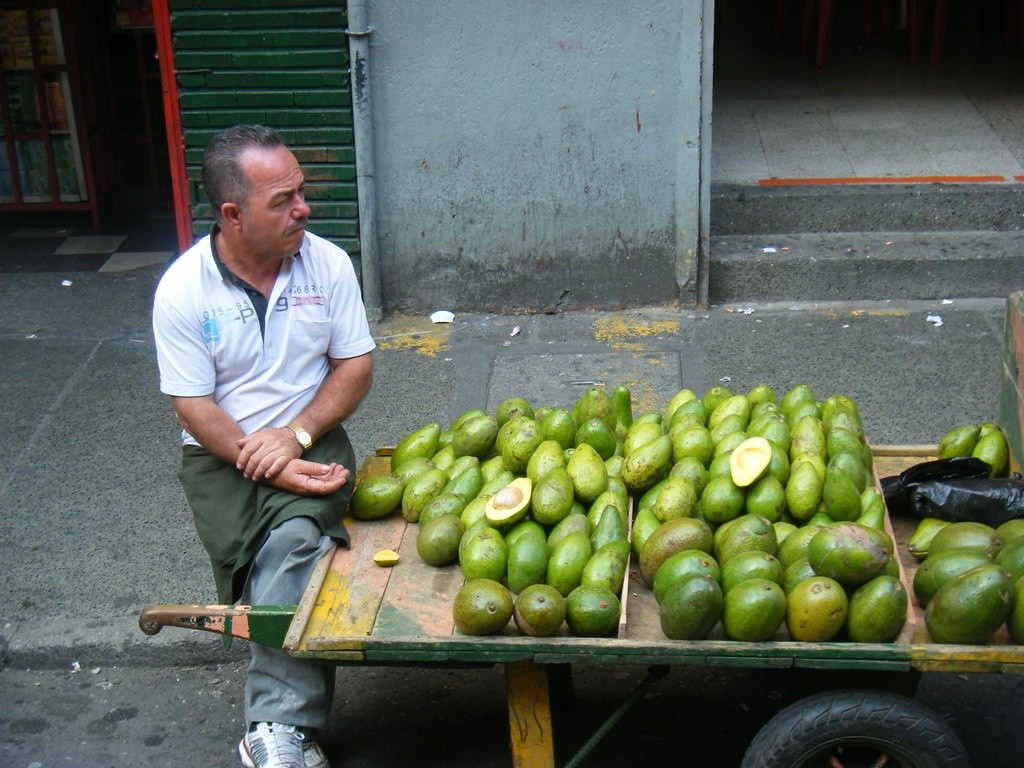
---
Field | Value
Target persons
[153,124,378,767]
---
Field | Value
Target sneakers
[238,718,332,768]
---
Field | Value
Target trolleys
[138,288,1024,766]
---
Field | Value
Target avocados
[348,386,1023,644]
